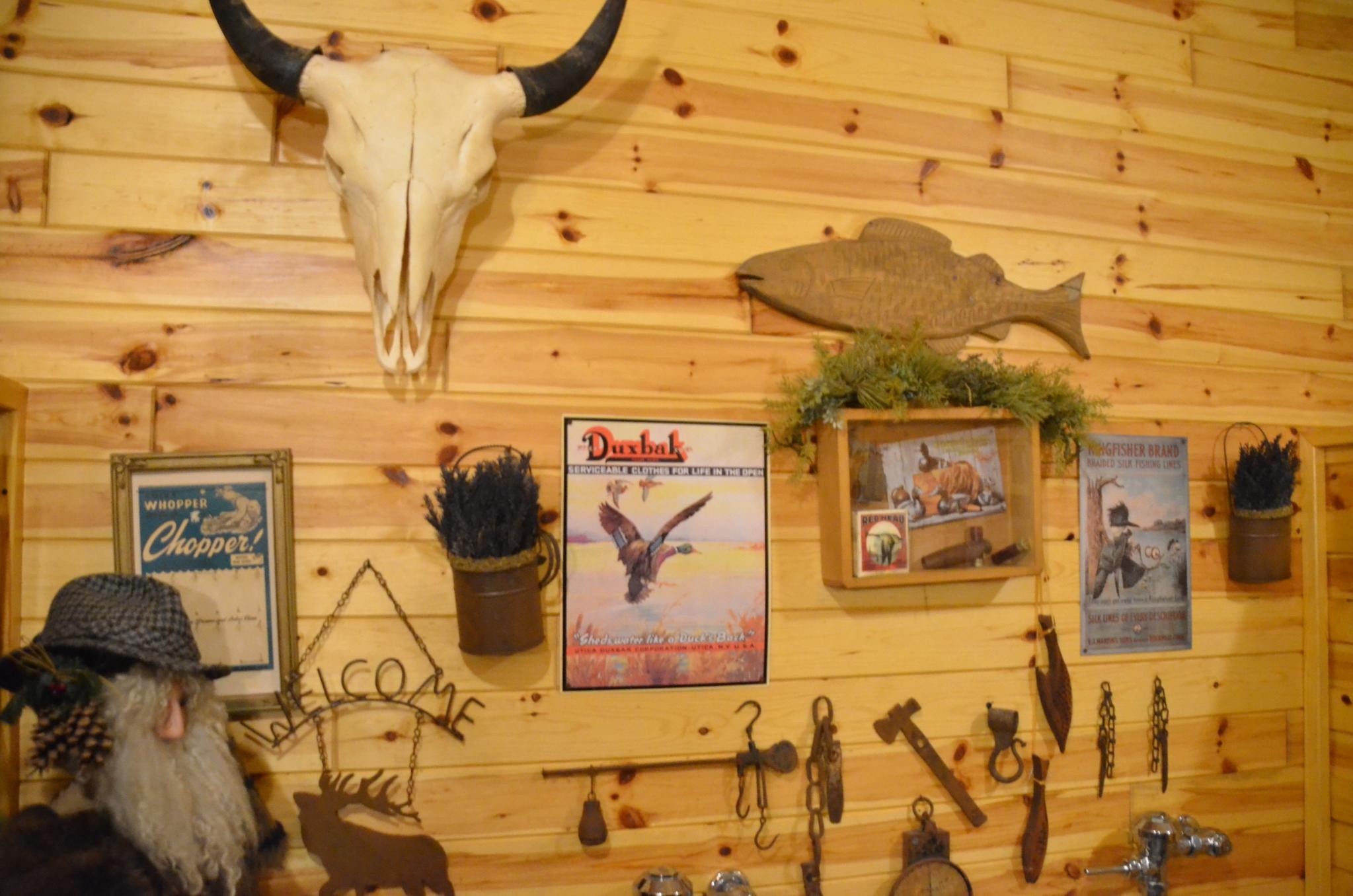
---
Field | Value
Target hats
[0,573,229,698]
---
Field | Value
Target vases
[1225,500,1294,583]
[444,541,546,658]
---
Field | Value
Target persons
[0,573,287,896]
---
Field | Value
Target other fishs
[737,216,1091,363]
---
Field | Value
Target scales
[542,740,797,850]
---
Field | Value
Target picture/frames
[110,446,299,716]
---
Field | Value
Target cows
[207,0,638,377]
